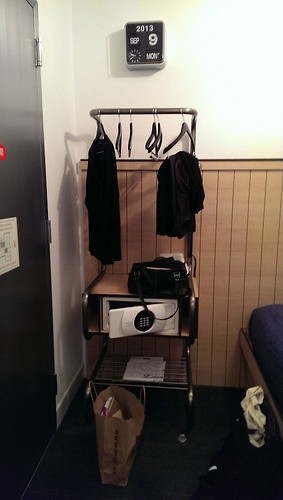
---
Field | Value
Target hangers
[162,107,196,155]
[88,108,115,152]
[127,108,134,158]
[145,107,158,159]
[148,108,163,156]
[114,107,123,159]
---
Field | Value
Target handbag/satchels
[86,379,146,488]
[126,257,189,321]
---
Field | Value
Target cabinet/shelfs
[79,105,205,443]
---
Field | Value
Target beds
[236,302,283,469]
